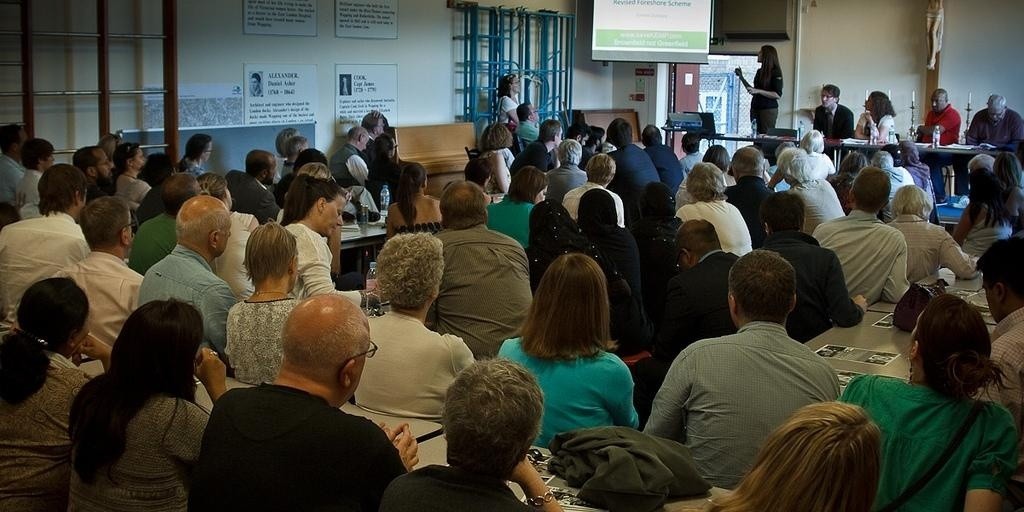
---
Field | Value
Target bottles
[888,125,895,144]
[364,262,386,316]
[796,121,804,141]
[750,118,757,139]
[932,125,940,149]
[379,184,390,216]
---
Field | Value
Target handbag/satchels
[890,277,950,333]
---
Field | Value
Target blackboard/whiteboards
[119,120,317,180]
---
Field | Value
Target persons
[0,70,1024,512]
[735,46,784,136]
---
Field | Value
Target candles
[863,89,868,102]
[967,92,970,104]
[912,91,916,104]
[888,91,891,102]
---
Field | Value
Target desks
[77,215,997,512]
[837,139,1022,171]
[661,123,839,175]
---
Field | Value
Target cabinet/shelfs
[386,123,478,201]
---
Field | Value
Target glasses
[977,283,996,299]
[336,338,379,377]
[673,250,683,269]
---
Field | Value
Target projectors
[668,112,703,128]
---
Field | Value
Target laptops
[683,111,725,136]
